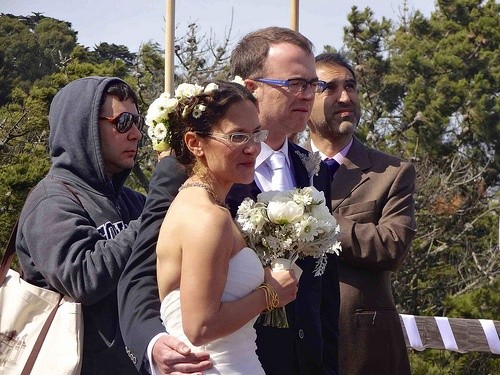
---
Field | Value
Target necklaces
[175,183,233,211]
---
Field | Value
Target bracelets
[258,282,281,314]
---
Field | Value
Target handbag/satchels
[0,180,85,375]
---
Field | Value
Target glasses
[253,78,327,93]
[194,130,268,145]
[98,112,145,134]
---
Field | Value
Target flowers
[144,83,219,153]
[295,149,322,186]
[230,75,246,86]
[234,185,343,328]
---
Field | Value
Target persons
[293,52,417,375]
[119,24,341,375]
[17,74,146,375]
[154,77,298,375]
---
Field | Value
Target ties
[324,158,340,184]
[264,151,290,192]
